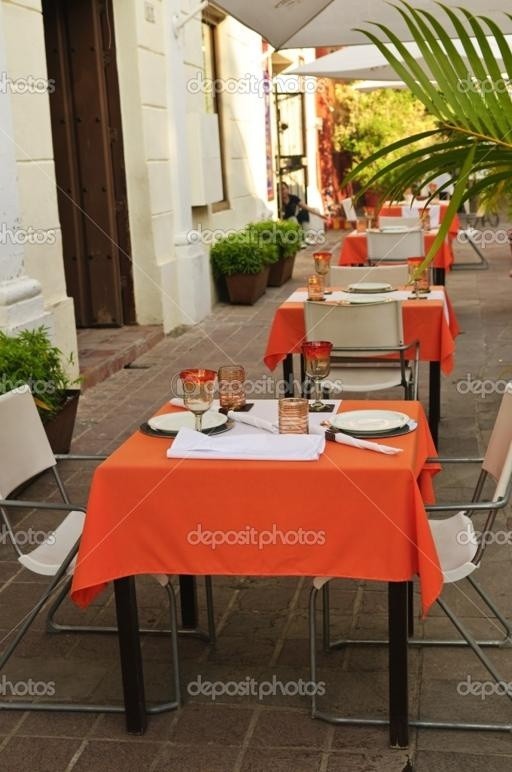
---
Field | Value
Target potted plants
[0,325,82,454]
[341,139,387,208]
[245,218,305,287]
[208,231,279,306]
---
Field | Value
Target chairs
[303,300,421,401]
[328,216,425,288]
[0,383,216,716]
[309,377,512,732]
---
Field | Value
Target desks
[338,228,453,285]
[372,199,459,240]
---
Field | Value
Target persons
[276,181,331,249]
[434,170,456,199]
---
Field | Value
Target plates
[146,410,228,436]
[349,281,391,291]
[330,408,409,434]
[347,294,388,304]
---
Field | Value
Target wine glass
[364,206,375,231]
[300,340,333,411]
[312,252,333,295]
[179,367,217,436]
[407,256,428,300]
[418,208,430,231]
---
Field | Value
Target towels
[166,398,342,462]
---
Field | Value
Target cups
[307,275,324,301]
[218,366,247,411]
[278,397,310,435]
[356,217,368,233]
[412,264,431,293]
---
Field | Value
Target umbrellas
[204,0,512,52]
[350,73,510,94]
[282,35,511,83]
